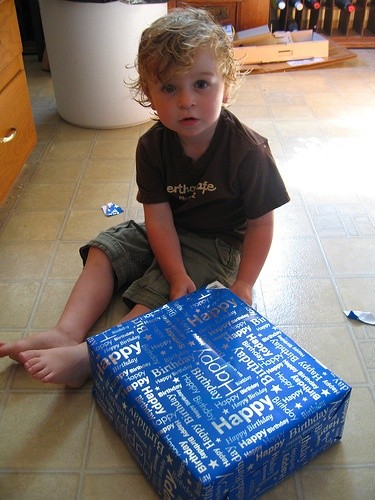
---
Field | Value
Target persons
[0,8,291,388]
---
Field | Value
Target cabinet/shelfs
[0,0,39,209]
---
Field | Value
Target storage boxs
[231,29,330,63]
[86,278,351,500]
